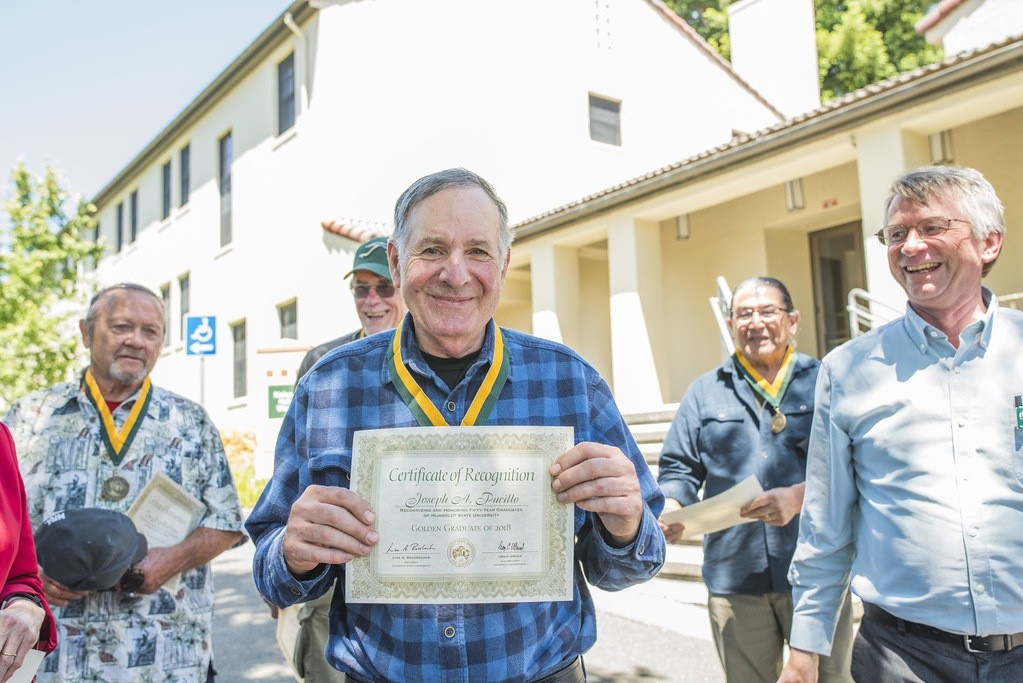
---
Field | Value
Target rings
[2,652,17,656]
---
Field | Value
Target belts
[860,600,1023,654]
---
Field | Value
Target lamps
[786,179,806,212]
[674,214,691,240]
[930,128,954,168]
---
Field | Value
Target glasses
[347,276,395,299]
[874,215,971,244]
[732,304,790,323]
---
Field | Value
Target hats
[33,506,148,592]
[342,237,393,282]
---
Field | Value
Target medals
[770,413,786,434]
[104,476,130,501]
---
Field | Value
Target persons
[277,236,409,683]
[6,283,249,683]
[243,166,667,683]
[778,168,1023,683]
[657,278,857,683]
[0,421,58,683]
